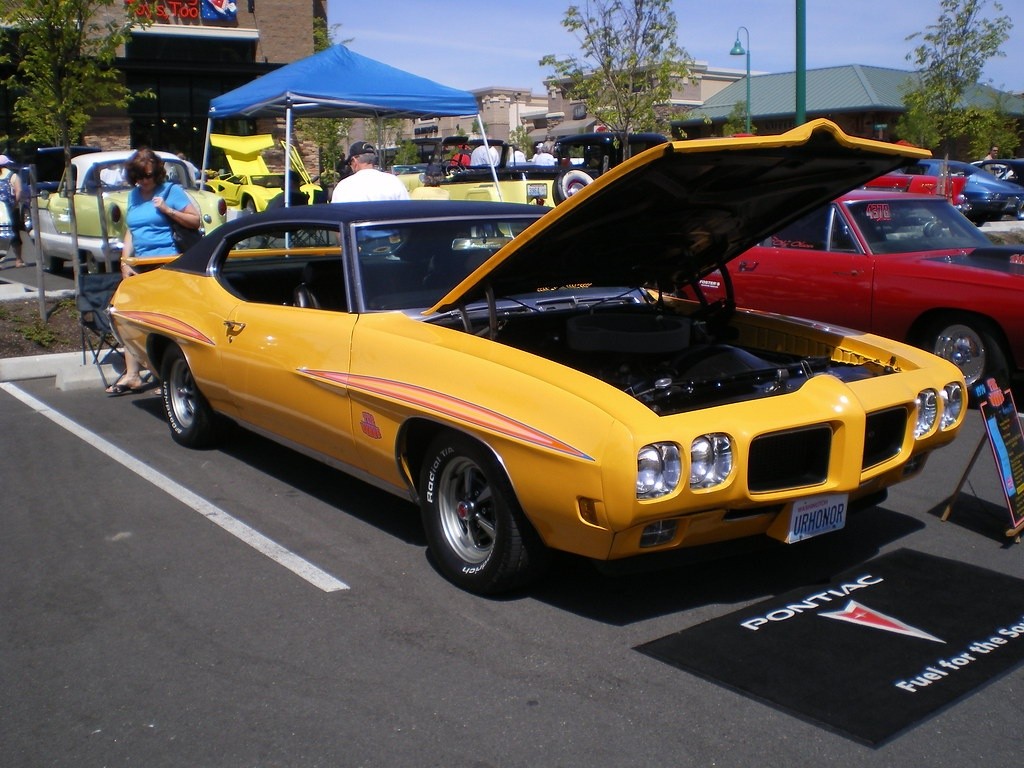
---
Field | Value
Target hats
[278,172,299,182]
[0,155,15,165]
[343,141,376,164]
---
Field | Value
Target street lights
[729,25,752,137]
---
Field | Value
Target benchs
[224,234,444,309]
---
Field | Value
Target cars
[101,118,969,598]
[897,159,1024,223]
[395,135,595,207]
[682,186,1024,409]
[976,159,1024,187]
[28,145,228,276]
[555,131,669,174]
[855,168,967,234]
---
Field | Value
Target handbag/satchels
[163,184,202,253]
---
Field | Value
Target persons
[536,141,555,165]
[450,143,471,167]
[0,155,27,267]
[105,149,200,393]
[99,164,127,187]
[265,171,302,211]
[331,142,409,254]
[410,164,449,201]
[510,145,526,163]
[983,145,998,175]
[471,135,501,167]
[532,143,543,163]
[168,153,200,186]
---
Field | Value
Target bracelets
[171,209,174,215]
[121,265,127,270]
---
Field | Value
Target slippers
[105,381,142,393]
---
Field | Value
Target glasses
[133,172,156,181]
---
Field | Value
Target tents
[199,45,515,242]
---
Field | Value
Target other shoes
[17,261,30,268]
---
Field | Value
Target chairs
[77,273,127,388]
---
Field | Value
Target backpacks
[0,172,17,208]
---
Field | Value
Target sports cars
[195,132,328,213]
